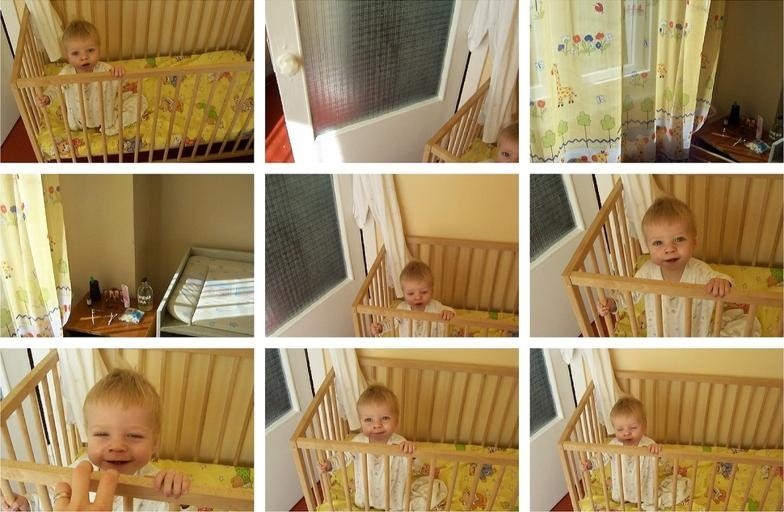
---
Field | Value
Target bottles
[137,278,153,311]
[768,117,782,146]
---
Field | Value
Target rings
[53,491,71,502]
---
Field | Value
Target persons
[598,196,734,337]
[53,459,121,512]
[370,261,456,336]
[39,18,148,137]
[317,384,415,512]
[2,366,200,512]
[496,122,518,163]
[581,396,663,506]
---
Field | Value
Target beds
[0,0,784,512]
